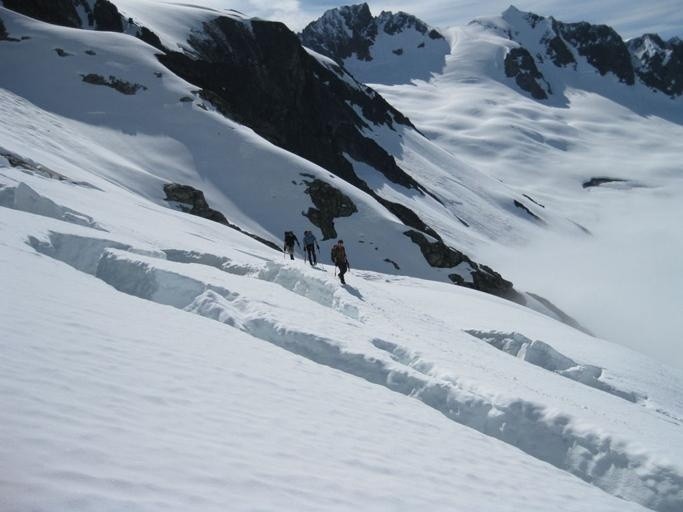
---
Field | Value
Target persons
[303,231,320,266]
[283,230,301,261]
[331,239,350,285]
